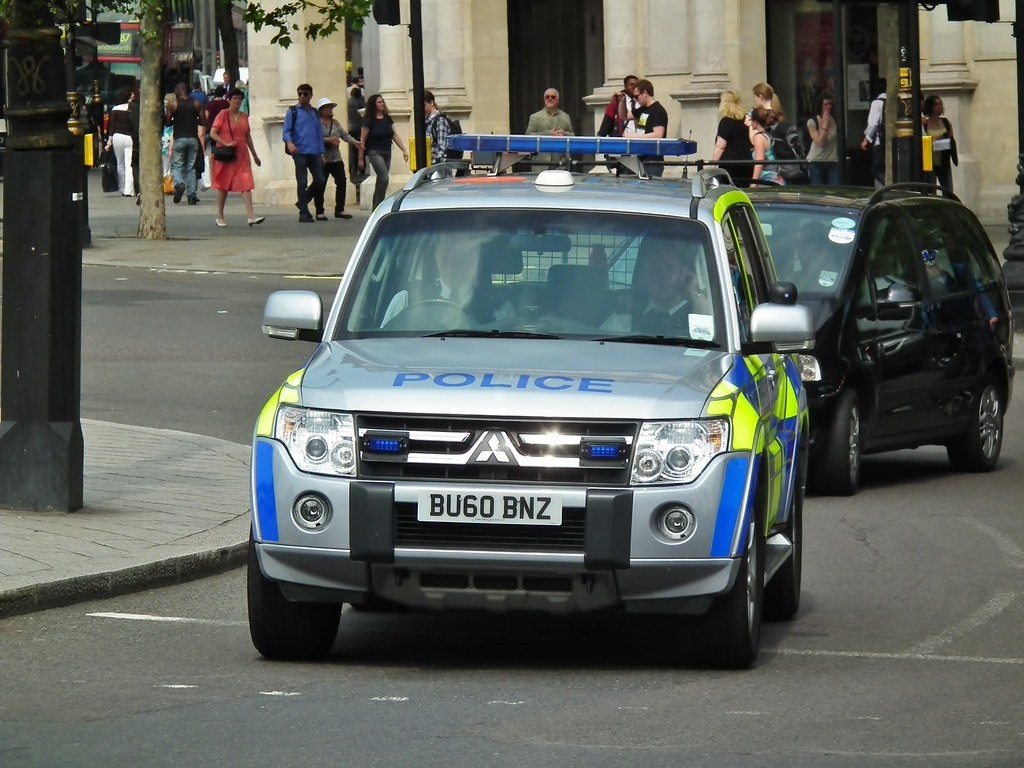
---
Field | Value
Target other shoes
[188,196,198,205]
[296,202,315,223]
[316,214,328,220]
[173,182,186,204]
[136,193,141,206]
[334,211,353,219]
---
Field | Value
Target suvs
[248,163,812,661]
[738,178,1012,496]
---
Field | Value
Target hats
[316,98,337,110]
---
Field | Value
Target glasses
[544,94,556,100]
[298,92,311,97]
[632,92,642,99]
[749,119,753,121]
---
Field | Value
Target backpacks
[752,121,812,182]
[875,97,906,155]
[431,115,464,160]
[802,115,818,157]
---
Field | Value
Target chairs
[547,264,612,329]
[465,235,523,326]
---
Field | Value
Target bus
[53,0,221,136]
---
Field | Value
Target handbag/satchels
[608,117,623,137]
[213,144,237,162]
[100,150,119,192]
[161,173,177,194]
[197,154,212,191]
[284,106,297,155]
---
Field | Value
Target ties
[445,287,461,316]
[630,98,637,113]
[224,84,228,92]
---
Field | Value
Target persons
[282,84,366,222]
[712,90,754,188]
[347,76,365,178]
[861,78,958,196]
[596,75,668,177]
[162,70,249,205]
[424,90,452,179]
[104,87,140,206]
[598,248,712,333]
[525,88,575,172]
[784,221,841,291]
[749,107,786,188]
[380,232,515,328]
[744,83,784,145]
[358,94,409,212]
[210,88,265,227]
[806,95,840,185]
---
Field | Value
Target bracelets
[748,125,752,127]
[822,128,827,130]
[694,292,701,298]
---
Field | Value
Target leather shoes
[215,216,227,227]
[247,216,265,227]
